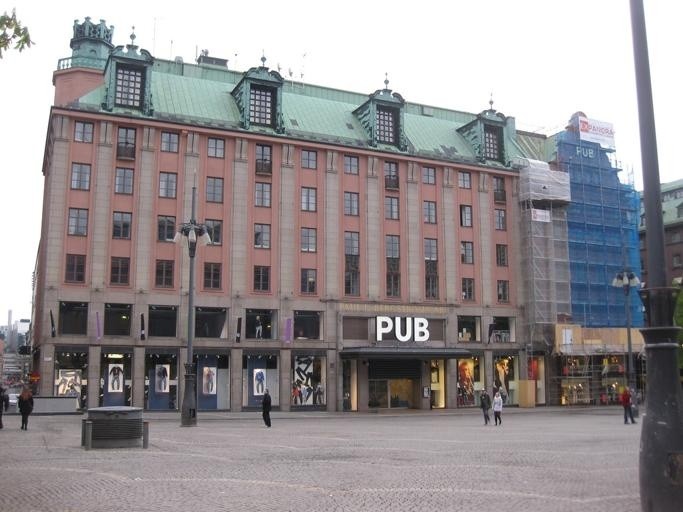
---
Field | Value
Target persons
[205,367,214,393]
[17,389,34,430]
[255,370,264,393]
[491,392,503,426]
[494,360,509,404]
[262,389,272,428]
[459,361,474,400]
[109,367,123,390]
[622,386,636,424]
[255,315,262,338]
[480,390,491,425]
[568,383,584,405]
[0,387,10,429]
[157,364,168,391]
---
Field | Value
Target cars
[8,393,21,408]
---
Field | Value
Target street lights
[172,167,211,427]
[612,270,642,417]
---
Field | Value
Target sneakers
[625,421,638,424]
[485,416,501,425]
[21,427,27,430]
